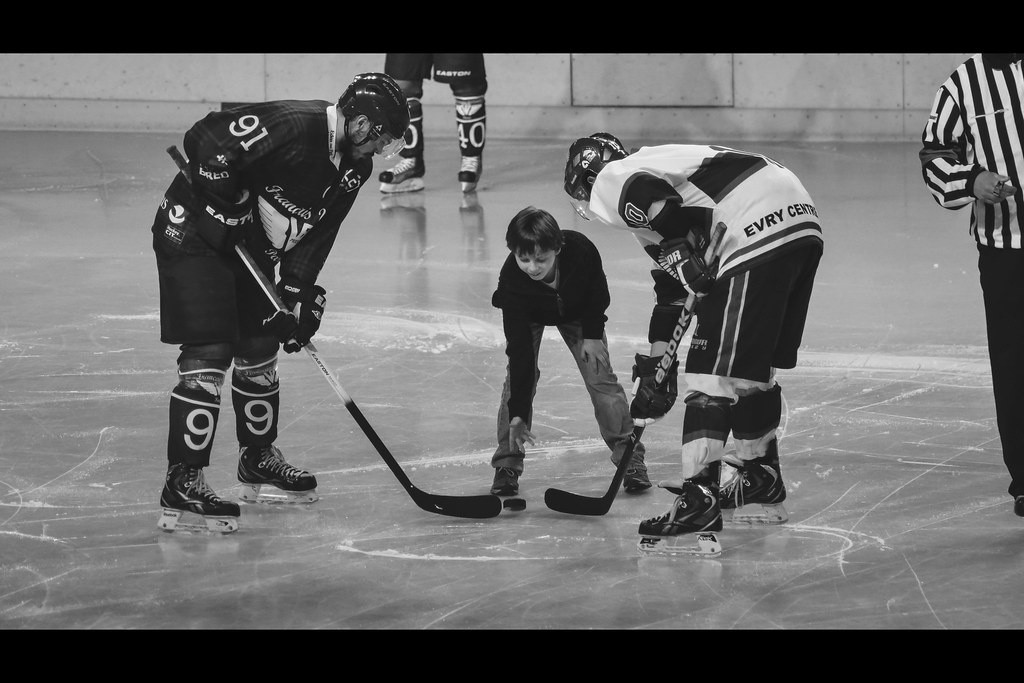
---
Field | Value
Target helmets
[563,132,631,220]
[339,73,410,160]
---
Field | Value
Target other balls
[503,498,527,511]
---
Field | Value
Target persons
[919,53,1024,517]
[490,207,652,497]
[380,53,488,195]
[563,132,824,558]
[150,72,410,536]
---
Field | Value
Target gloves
[629,354,678,426]
[262,276,327,353]
[659,236,720,296]
[196,184,259,254]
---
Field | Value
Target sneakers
[490,467,519,495]
[156,460,240,534]
[623,468,652,493]
[380,152,425,193]
[717,434,789,524]
[457,154,482,193]
[639,479,724,558]
[237,442,318,503]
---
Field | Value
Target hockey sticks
[543,222,729,516]
[165,144,502,519]
